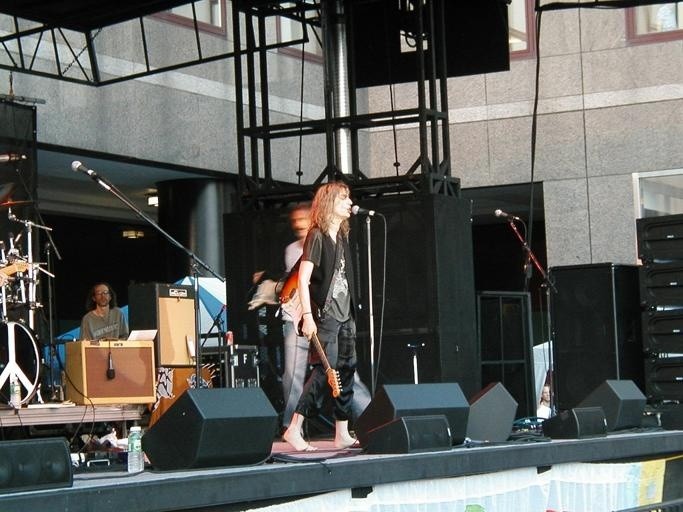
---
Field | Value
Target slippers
[281,443,319,455]
[335,439,361,450]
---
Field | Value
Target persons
[251,200,316,442]
[76,283,129,342]
[281,182,363,454]
[535,383,558,424]
[0,271,11,287]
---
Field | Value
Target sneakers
[97,431,117,448]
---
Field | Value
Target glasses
[95,291,109,295]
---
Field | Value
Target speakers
[366,415,452,453]
[544,407,608,440]
[64,339,158,404]
[0,436,73,494]
[550,263,645,415]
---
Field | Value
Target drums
[0,322,42,408]
[0,264,29,310]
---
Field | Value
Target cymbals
[0,200,36,209]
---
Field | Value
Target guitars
[276,255,343,397]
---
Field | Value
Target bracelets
[303,311,312,317]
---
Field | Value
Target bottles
[9,377,22,409]
[128,426,145,472]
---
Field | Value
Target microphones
[352,205,375,217]
[106,353,115,378]
[71,161,97,179]
[0,154,27,163]
[494,208,520,221]
